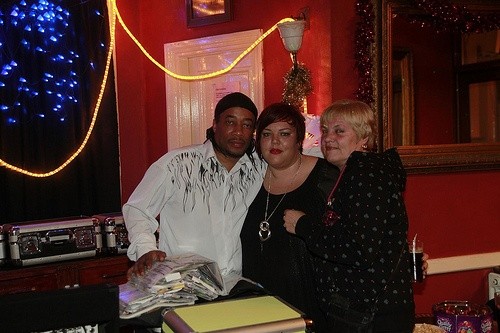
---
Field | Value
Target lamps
[277,21,305,75]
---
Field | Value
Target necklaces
[259,154,302,242]
[320,166,346,227]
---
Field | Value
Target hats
[215,92,258,121]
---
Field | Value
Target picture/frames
[185,0,233,28]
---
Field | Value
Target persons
[239,103,429,333]
[121,92,268,281]
[283,100,416,333]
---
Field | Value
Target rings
[283,223,286,228]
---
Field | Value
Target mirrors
[370,0,500,176]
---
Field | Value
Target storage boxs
[433,301,493,333]
[161,293,313,333]
[0,213,160,269]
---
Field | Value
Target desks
[0,253,135,295]
